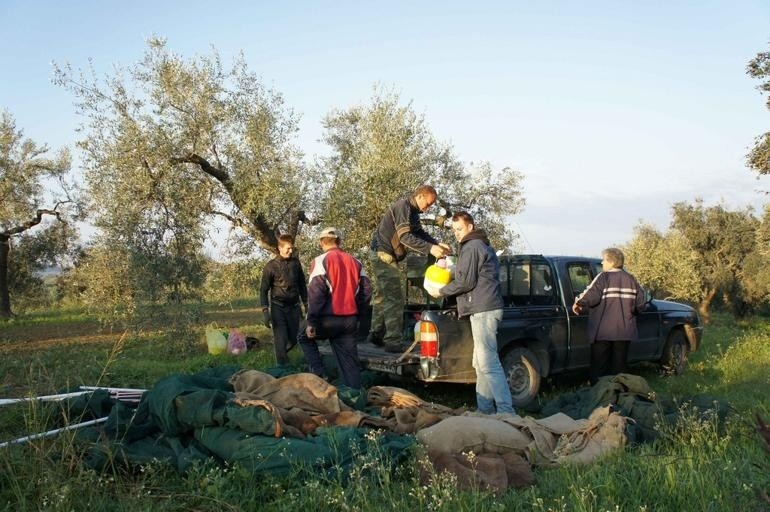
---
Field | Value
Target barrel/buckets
[423,258,454,300]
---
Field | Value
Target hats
[319,227,340,239]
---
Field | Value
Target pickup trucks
[296,252,705,413]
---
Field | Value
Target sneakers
[372,339,387,348]
[385,344,414,353]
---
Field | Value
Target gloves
[264,312,273,328]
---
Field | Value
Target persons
[571,248,647,388]
[260,233,309,366]
[296,225,372,390]
[438,212,515,415]
[367,185,452,352]
